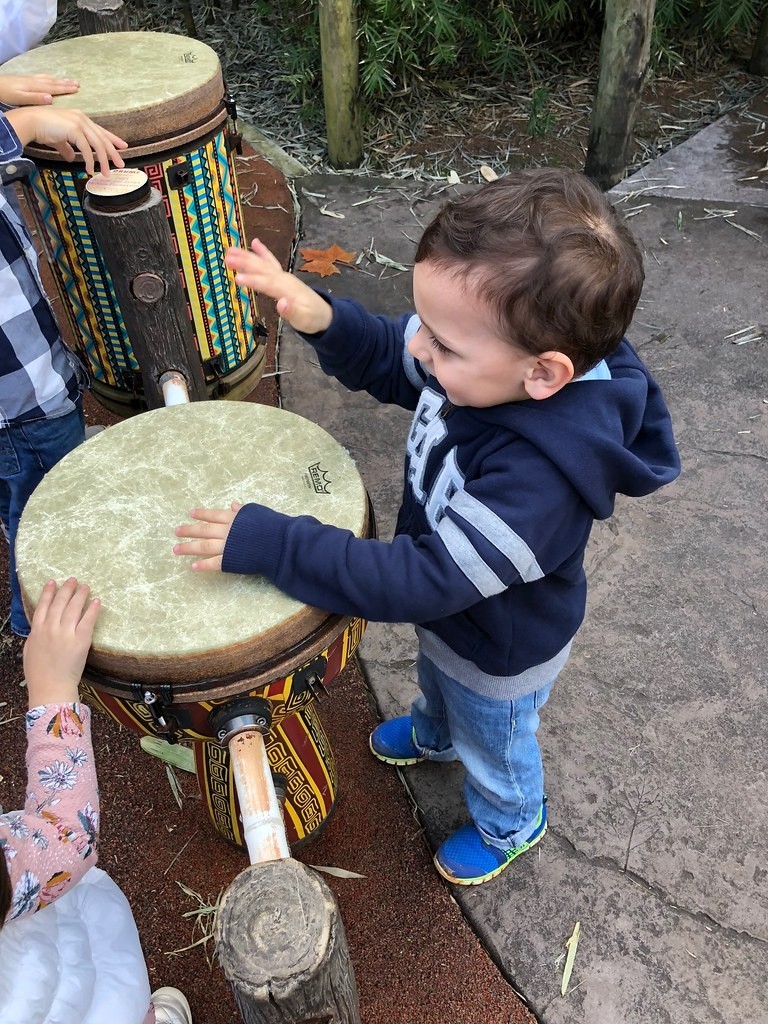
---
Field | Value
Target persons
[0,0,131,640]
[173,164,685,886]
[0,576,193,1024]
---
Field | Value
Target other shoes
[149,987,193,1024]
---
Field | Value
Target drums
[14,400,378,857]
[0,31,267,417]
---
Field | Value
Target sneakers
[433,793,547,885]
[368,717,463,766]
